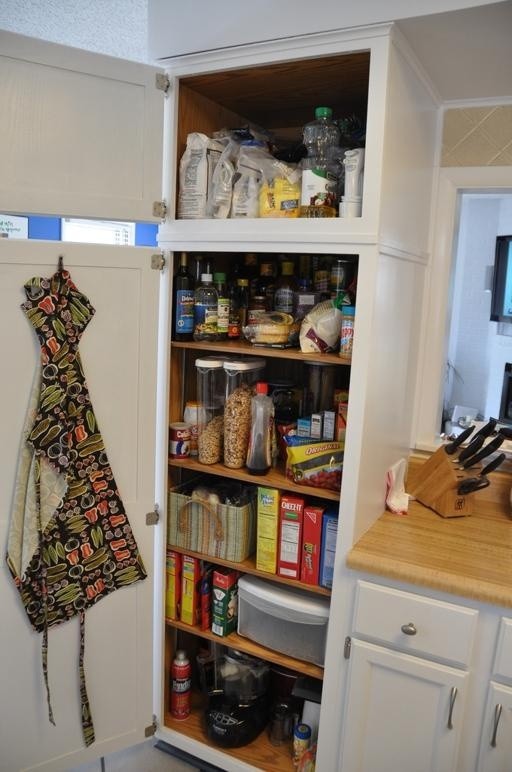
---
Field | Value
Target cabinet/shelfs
[341,561,512,772]
[151,23,442,771]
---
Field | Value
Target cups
[268,702,295,746]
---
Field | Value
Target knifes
[445,420,505,476]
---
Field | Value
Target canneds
[170,422,192,459]
[248,294,265,323]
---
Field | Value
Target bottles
[194,254,256,341]
[184,400,206,456]
[195,356,266,470]
[339,305,355,359]
[277,391,299,461]
[297,108,342,217]
[173,252,194,341]
[256,261,311,320]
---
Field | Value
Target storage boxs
[233,576,328,669]
[169,476,256,563]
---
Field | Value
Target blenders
[203,645,276,748]
